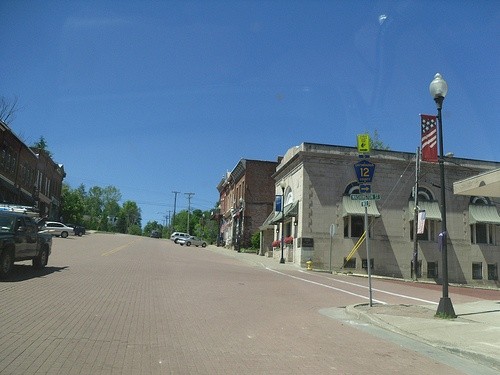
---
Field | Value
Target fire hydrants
[305,258,314,271]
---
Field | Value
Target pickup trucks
[0,209,53,277]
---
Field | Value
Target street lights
[428,72,459,320]
[278,179,287,265]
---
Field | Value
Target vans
[170,231,191,245]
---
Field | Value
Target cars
[151,230,160,238]
[177,235,209,248]
[65,223,86,237]
[37,221,75,239]
